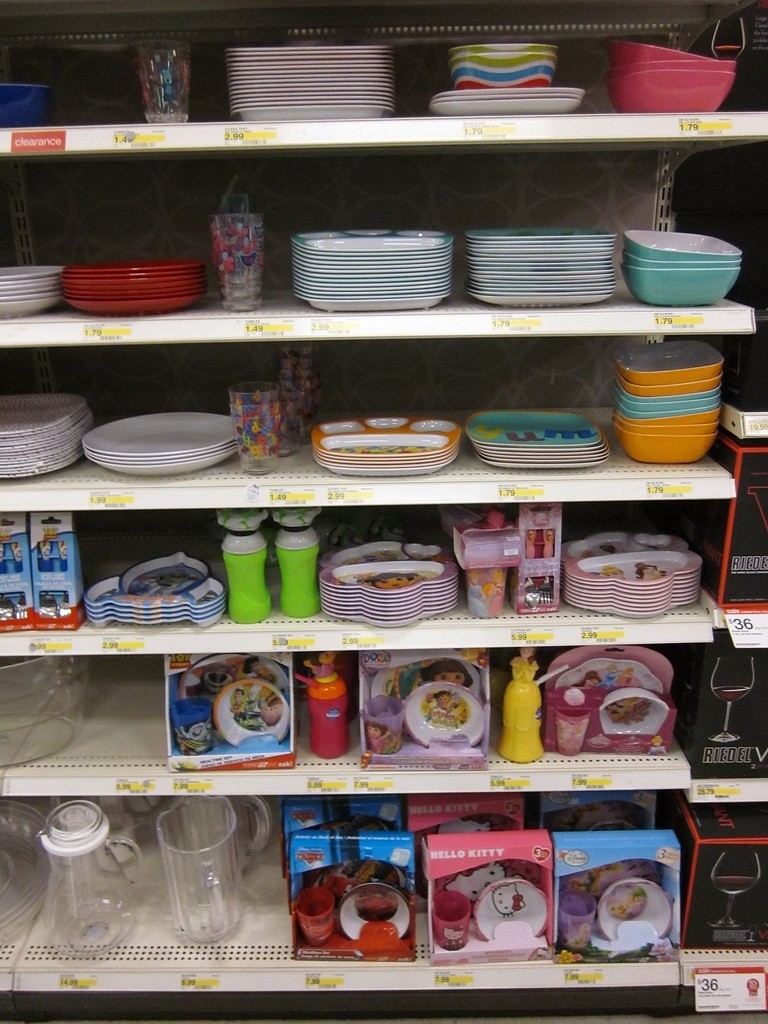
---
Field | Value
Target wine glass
[709,654,756,743]
[708,850,760,931]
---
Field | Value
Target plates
[222,46,397,121]
[82,531,703,635]
[430,84,587,116]
[0,225,621,319]
[0,391,609,476]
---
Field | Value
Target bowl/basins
[0,83,53,129]
[403,682,484,749]
[338,884,411,940]
[473,878,548,942]
[0,656,83,769]
[0,799,52,942]
[211,677,289,748]
[620,229,743,305]
[598,879,672,942]
[601,685,669,734]
[612,339,725,464]
[449,36,737,112]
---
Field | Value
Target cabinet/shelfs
[0,0,45,1024]
[15,0,768,1024]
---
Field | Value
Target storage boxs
[442,502,563,614]
[691,430,768,610]
[162,630,768,778]
[0,512,86,631]
[280,791,768,955]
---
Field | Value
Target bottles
[293,649,351,758]
[215,508,520,624]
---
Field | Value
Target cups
[555,706,590,758]
[207,195,266,312]
[170,698,214,757]
[432,890,472,950]
[230,345,325,475]
[711,16,746,63]
[132,37,192,125]
[294,884,334,949]
[364,695,406,755]
[557,887,597,949]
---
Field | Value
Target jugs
[37,796,271,954]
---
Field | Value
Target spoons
[524,576,539,611]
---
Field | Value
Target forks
[539,575,553,607]
